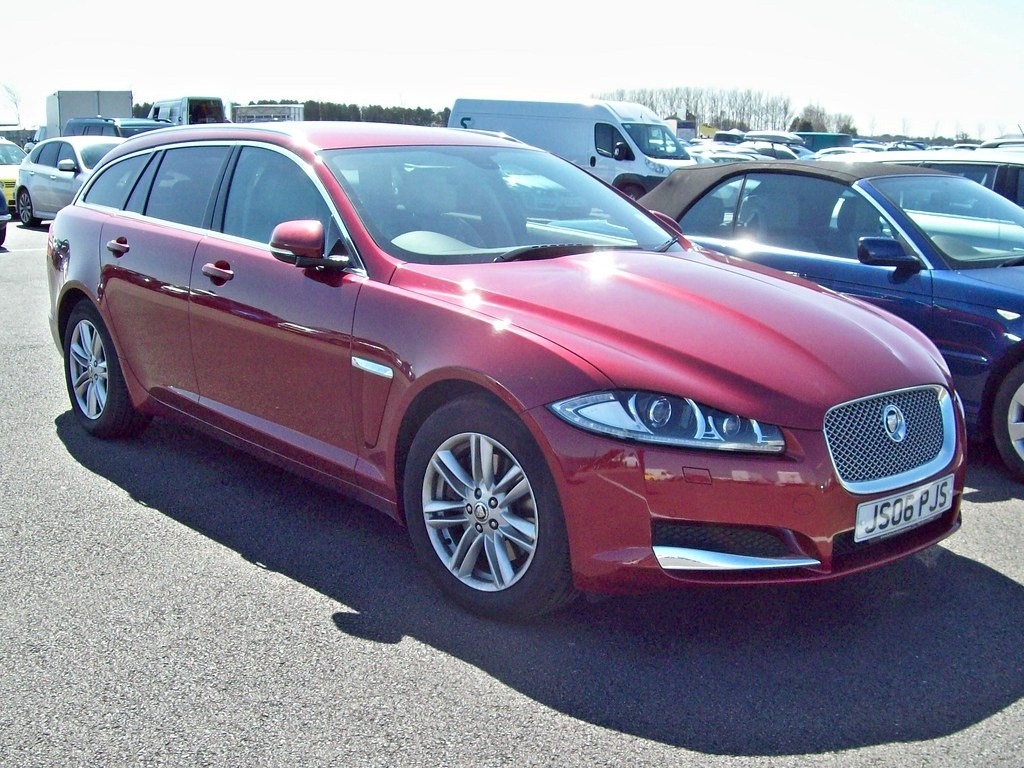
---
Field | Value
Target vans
[63,117,177,139]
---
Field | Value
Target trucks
[146,97,233,126]
[446,98,697,201]
[24,90,133,154]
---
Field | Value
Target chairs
[740,193,821,255]
[680,196,733,240]
[386,168,486,250]
[834,197,893,259]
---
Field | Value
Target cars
[0,136,28,219]
[544,159,1024,485]
[649,130,1024,168]
[14,136,129,227]
[817,148,1024,255]
[0,191,12,246]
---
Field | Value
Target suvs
[46,120,969,626]
